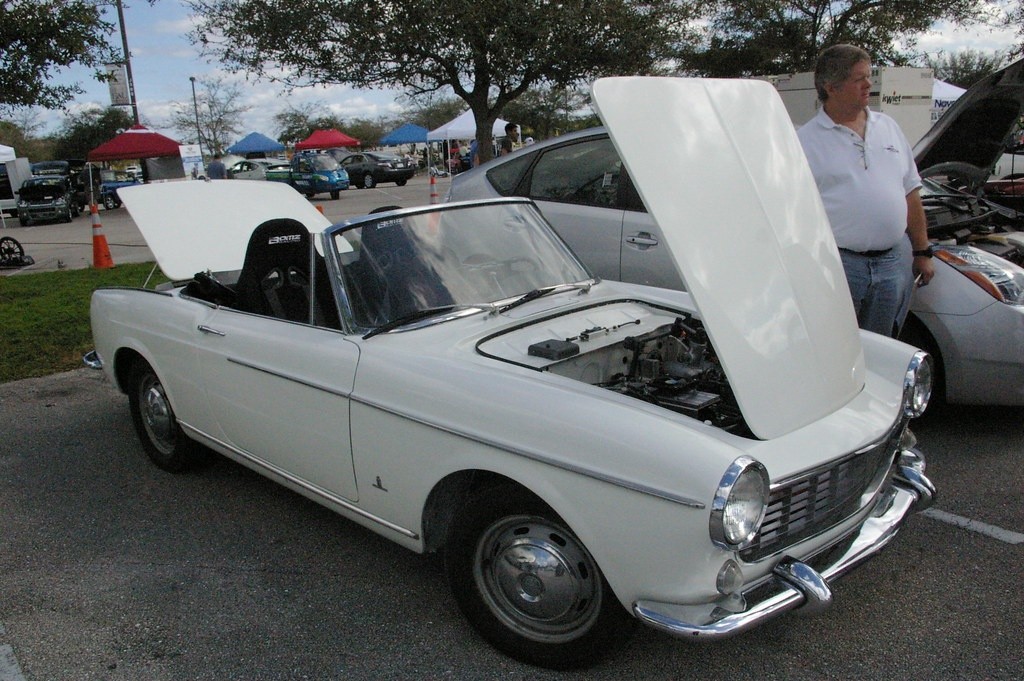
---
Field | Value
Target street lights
[188,77,205,167]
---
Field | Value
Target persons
[405,144,428,164]
[797,44,935,339]
[442,122,519,174]
[207,152,228,179]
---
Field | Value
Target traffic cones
[89,206,116,269]
[427,176,442,234]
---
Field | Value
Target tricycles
[266,148,350,200]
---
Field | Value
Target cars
[13,174,80,226]
[437,54,1024,420]
[225,157,291,181]
[30,160,145,211]
[339,152,414,189]
[322,148,350,164]
[80,71,937,672]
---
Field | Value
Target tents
[225,132,285,154]
[88,122,185,169]
[295,129,360,149]
[380,108,520,144]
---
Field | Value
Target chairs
[233,205,457,329]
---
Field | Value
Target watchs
[912,245,933,258]
